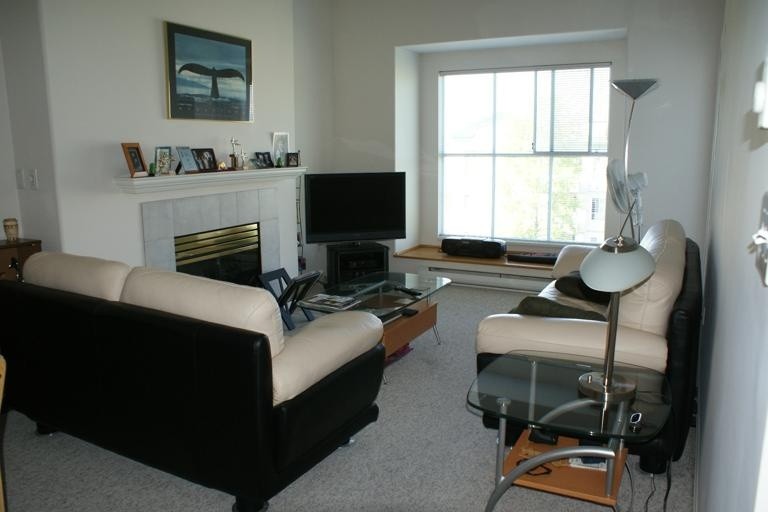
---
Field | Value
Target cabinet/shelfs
[0,237,42,284]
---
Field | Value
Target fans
[606,160,649,244]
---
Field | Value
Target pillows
[507,297,609,321]
[556,271,608,304]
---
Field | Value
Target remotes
[394,284,423,296]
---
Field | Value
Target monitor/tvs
[304,172,405,244]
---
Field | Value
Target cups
[3,218,19,242]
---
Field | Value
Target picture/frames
[165,21,252,123]
[256,152,273,167]
[122,142,149,177]
[175,146,200,173]
[272,132,289,167]
[288,153,298,167]
[156,148,171,175]
[191,149,217,170]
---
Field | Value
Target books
[297,293,361,312]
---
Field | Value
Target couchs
[0,251,385,511]
[477,218,701,474]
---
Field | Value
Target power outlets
[751,193,768,286]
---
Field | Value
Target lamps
[575,238,657,400]
